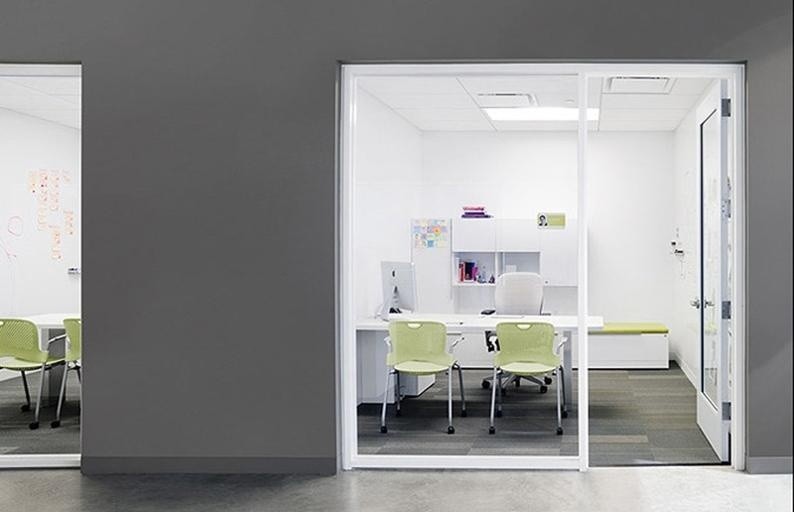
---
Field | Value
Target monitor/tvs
[374,261,418,322]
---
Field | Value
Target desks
[17,313,81,422]
[356,313,604,414]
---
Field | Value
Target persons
[538,215,548,226]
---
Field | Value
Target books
[461,205,491,218]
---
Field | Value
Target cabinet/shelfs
[408,216,579,371]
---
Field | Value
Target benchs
[588,323,669,369]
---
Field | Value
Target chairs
[0,319,66,430]
[488,320,568,436]
[380,318,466,435]
[49,317,81,429]
[480,272,551,394]
